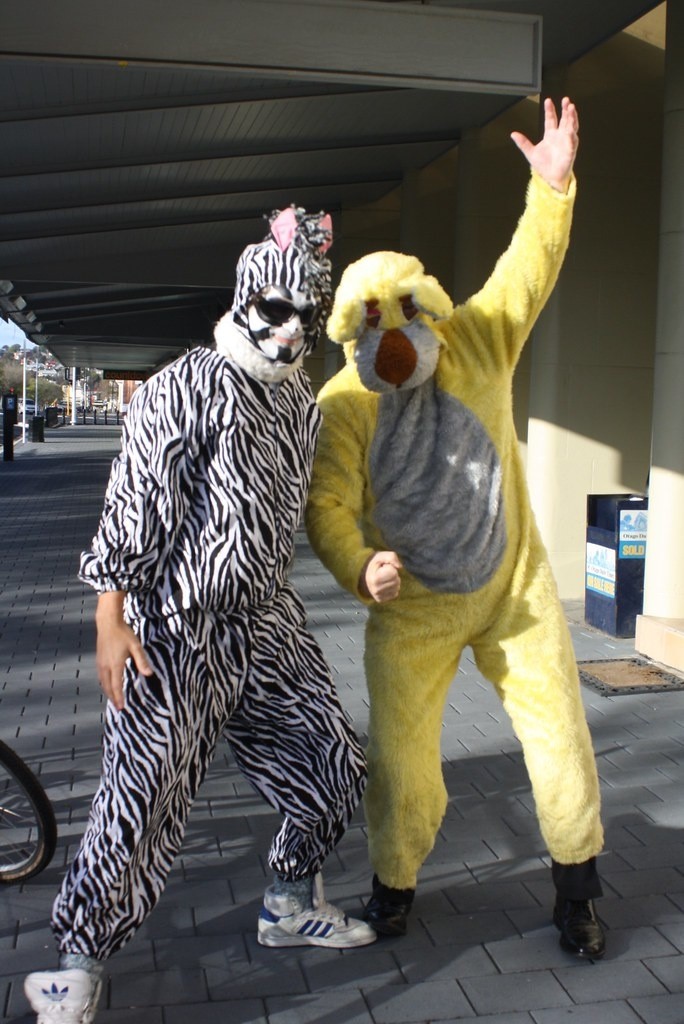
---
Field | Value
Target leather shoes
[552,890,606,959]
[364,871,414,938]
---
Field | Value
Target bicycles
[0,736,59,883]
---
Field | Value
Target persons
[305,96,608,960]
[24,205,379,1024]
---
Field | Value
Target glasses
[251,294,320,333]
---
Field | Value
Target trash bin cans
[29,415,45,442]
[584,492,649,639]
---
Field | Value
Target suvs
[17,398,39,414]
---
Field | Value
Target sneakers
[256,871,378,947]
[23,970,102,1024]
